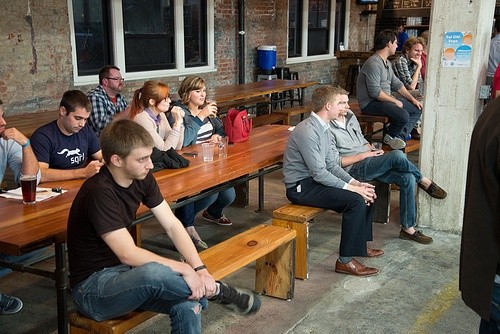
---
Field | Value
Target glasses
[163,94,170,102]
[107,77,124,83]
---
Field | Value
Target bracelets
[194,264,207,272]
[21,138,31,147]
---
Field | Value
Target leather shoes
[335,258,380,276]
[357,248,384,257]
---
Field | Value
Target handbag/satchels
[225,109,252,143]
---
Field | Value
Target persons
[0,99,41,315]
[130,80,208,264]
[329,84,447,245]
[282,85,385,277]
[458,16,500,334]
[167,75,236,226]
[84,65,133,139]
[356,21,429,153]
[30,90,106,184]
[67,118,262,334]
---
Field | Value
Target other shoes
[417,179,447,199]
[400,228,433,243]
[382,134,406,150]
[410,128,420,140]
[407,134,411,140]
[191,237,208,248]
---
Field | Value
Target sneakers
[208,280,261,315]
[202,210,232,225]
[0,292,23,314]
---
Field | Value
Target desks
[347,104,388,146]
[169,79,318,123]
[2,110,62,138]
[0,124,297,334]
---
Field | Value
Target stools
[256,68,301,115]
[345,63,363,94]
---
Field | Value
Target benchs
[65,101,422,334]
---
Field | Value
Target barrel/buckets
[257,45,276,71]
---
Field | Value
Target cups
[202,143,215,162]
[217,136,228,159]
[372,142,382,151]
[20,172,37,204]
